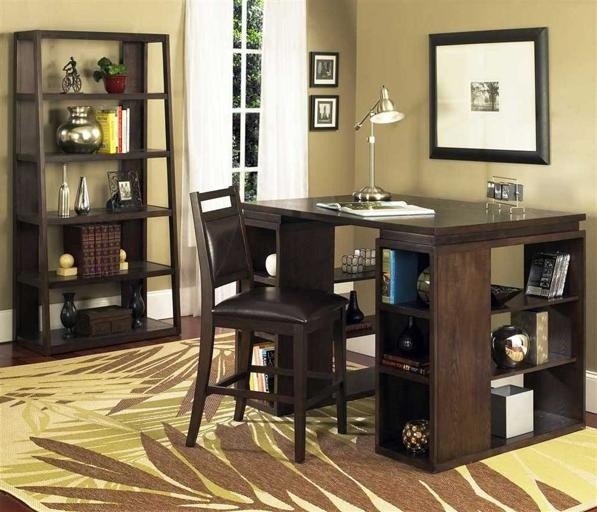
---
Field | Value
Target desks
[240,198,588,480]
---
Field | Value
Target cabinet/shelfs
[4,30,186,358]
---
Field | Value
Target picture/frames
[428,26,550,166]
[309,51,339,88]
[311,95,339,132]
[104,171,145,214]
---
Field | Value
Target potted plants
[94,54,128,93]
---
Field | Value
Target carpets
[1,331,596,512]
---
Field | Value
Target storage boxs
[490,384,535,440]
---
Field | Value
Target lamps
[350,84,403,201]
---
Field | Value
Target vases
[345,290,364,322]
[489,324,530,369]
[74,176,93,214]
[127,283,147,328]
[60,290,81,340]
[398,317,423,355]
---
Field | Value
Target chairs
[184,184,351,464]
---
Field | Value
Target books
[525,252,570,298]
[250,342,276,394]
[382,353,429,375]
[382,249,419,305]
[95,106,130,153]
[64,224,121,276]
[316,201,434,216]
